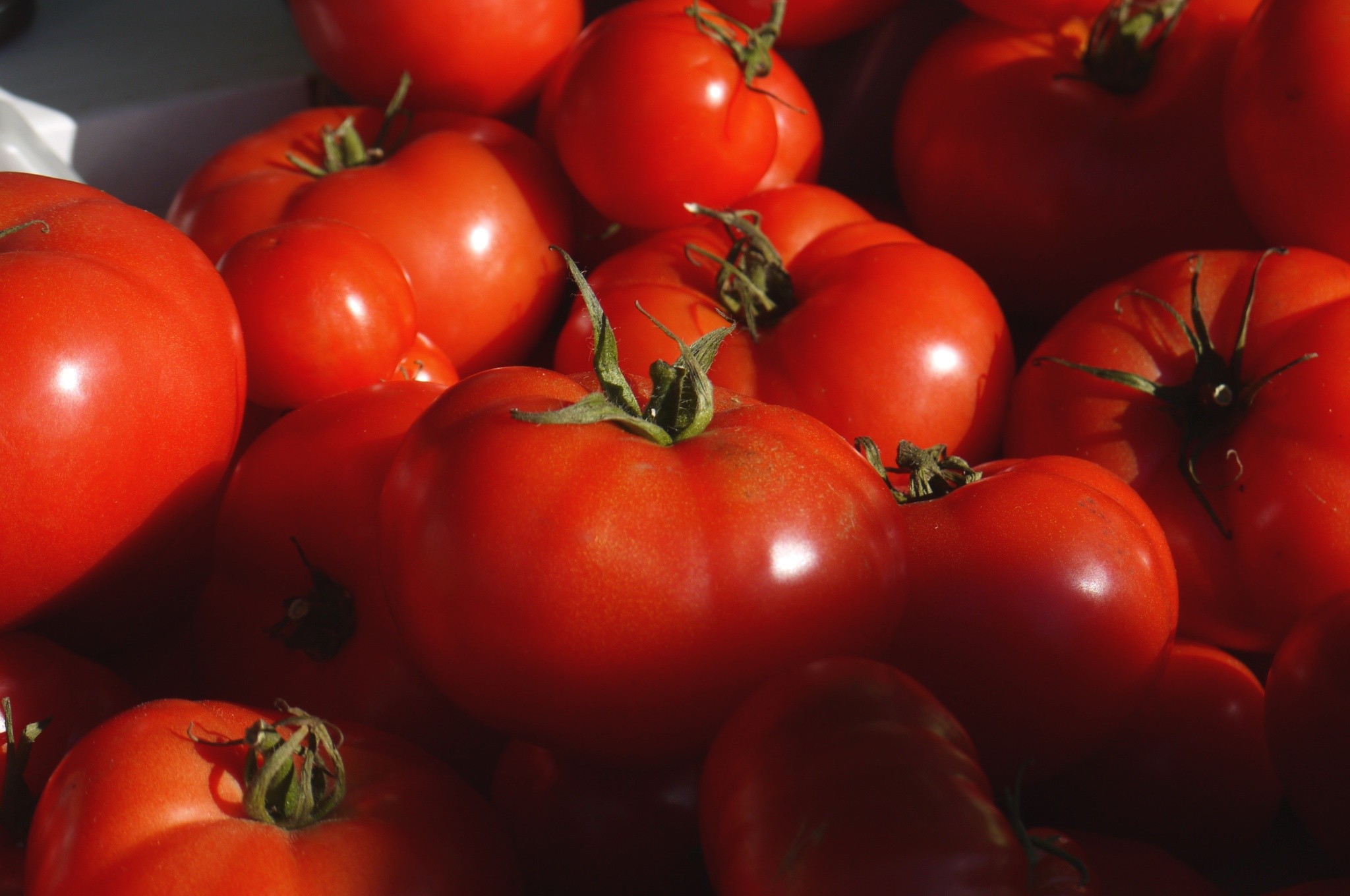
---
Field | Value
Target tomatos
[4,1,1350,895]
